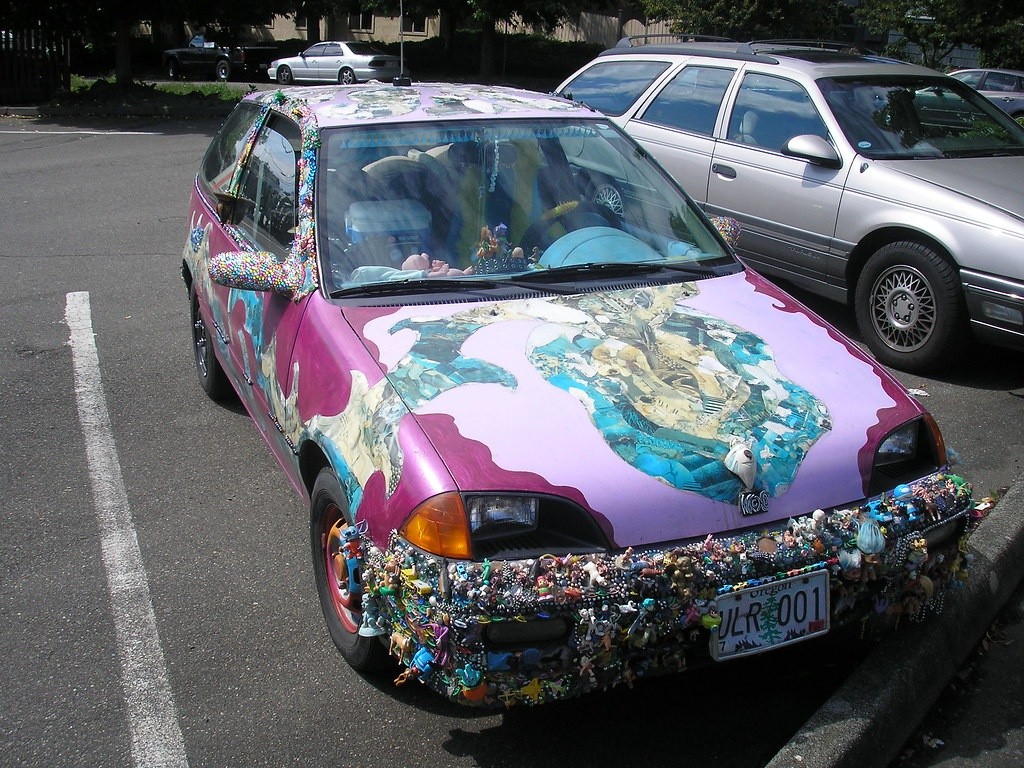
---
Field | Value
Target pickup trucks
[163,30,278,81]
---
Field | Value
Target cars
[878,67,1024,133]
[267,40,406,86]
[179,68,981,710]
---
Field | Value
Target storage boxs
[343,198,433,273]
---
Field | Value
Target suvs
[549,33,1024,373]
